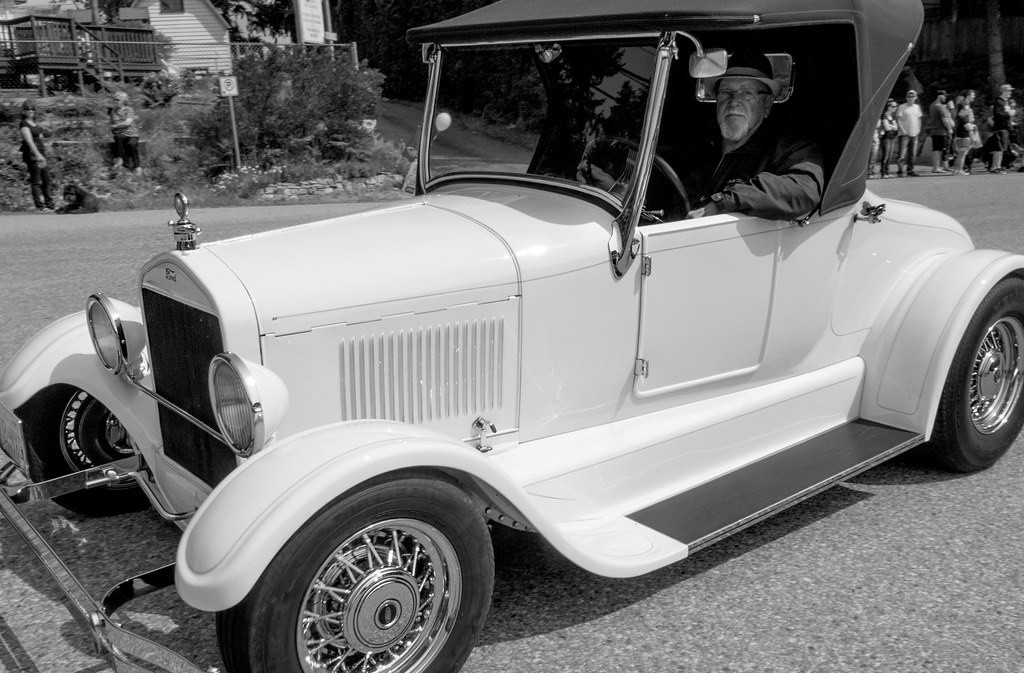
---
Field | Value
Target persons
[866,84,1018,175]
[109,91,142,175]
[20,100,60,212]
[578,52,826,222]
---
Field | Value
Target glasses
[716,88,771,103]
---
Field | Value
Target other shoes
[953,170,969,176]
[908,171,918,176]
[990,167,1006,174]
[889,174,894,178]
[932,169,946,172]
[880,175,889,179]
[868,175,877,179]
[945,168,952,171]
[897,173,902,178]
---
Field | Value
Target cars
[1,0,1024,673]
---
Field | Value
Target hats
[937,90,949,95]
[907,90,916,95]
[999,85,1015,91]
[703,50,783,97]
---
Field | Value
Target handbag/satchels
[973,130,983,148]
[954,137,972,150]
